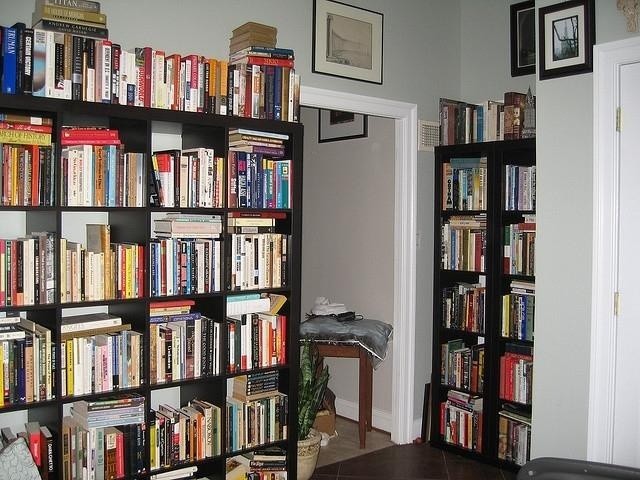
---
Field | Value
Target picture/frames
[539,0,595,81]
[311,0,384,85]
[509,0,537,77]
[317,107,368,142]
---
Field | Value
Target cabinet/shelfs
[0,93,302,480]
[427,137,535,475]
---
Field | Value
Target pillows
[301,314,393,361]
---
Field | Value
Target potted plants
[297,339,329,480]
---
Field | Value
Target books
[440,339,484,453]
[442,275,485,333]
[504,164,537,276]
[150,381,287,479]
[441,156,486,273]
[0,121,291,208]
[497,344,533,468]
[502,280,536,341]
[1,311,147,479]
[439,92,536,146]
[1,212,289,307]
[150,298,286,385]
[1,0,300,124]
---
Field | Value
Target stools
[300,342,372,448]
